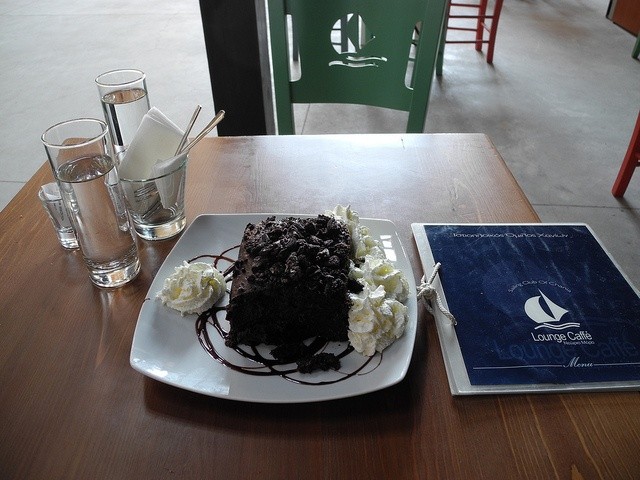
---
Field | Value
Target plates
[129,213,417,404]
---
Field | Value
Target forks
[140,104,202,222]
[133,110,225,202]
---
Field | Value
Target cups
[95,68,150,167]
[38,181,80,249]
[40,117,141,289]
[116,144,189,241]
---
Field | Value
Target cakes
[157,202,410,386]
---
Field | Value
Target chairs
[448,0,503,64]
[612,111,640,198]
[268,1,448,135]
